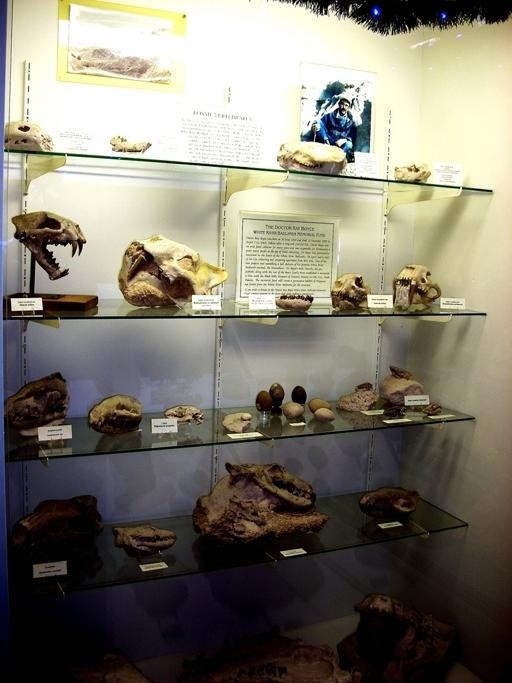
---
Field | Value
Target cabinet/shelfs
[5,150,494,591]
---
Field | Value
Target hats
[339,95,351,104]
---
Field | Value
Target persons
[310,98,355,152]
